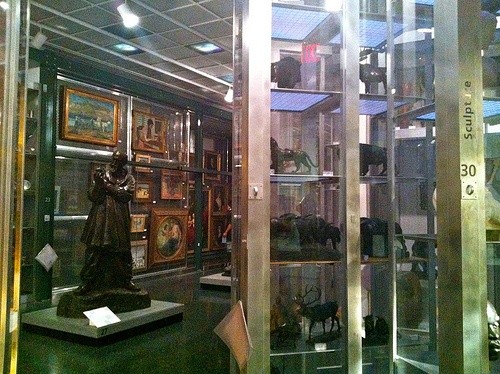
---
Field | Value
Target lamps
[117,0,139,28]
[223,86,234,103]
[30,28,48,50]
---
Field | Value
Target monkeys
[362,315,390,345]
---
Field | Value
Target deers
[294,284,340,340]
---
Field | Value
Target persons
[146,119,159,144]
[137,126,158,149]
[77,143,142,295]
[221,203,232,276]
[159,221,183,256]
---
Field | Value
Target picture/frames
[211,213,227,250]
[187,189,210,253]
[160,168,183,199]
[130,215,146,232]
[203,150,221,180]
[65,189,81,215]
[53,186,61,214]
[135,154,151,173]
[212,184,227,215]
[130,240,147,272]
[181,153,196,184]
[133,180,153,201]
[132,110,166,153]
[149,208,188,265]
[62,89,119,147]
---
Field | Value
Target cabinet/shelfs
[270,0,500,374]
[8,58,42,305]
